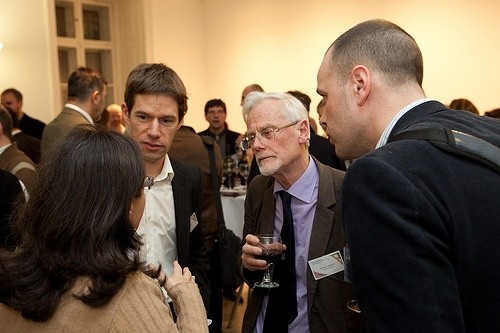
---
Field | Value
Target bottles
[222,152,234,190]
[237,150,250,186]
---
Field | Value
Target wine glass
[254,234,283,288]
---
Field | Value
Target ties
[280,192,301,322]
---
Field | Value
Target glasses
[239,117,301,150]
[133,176,157,199]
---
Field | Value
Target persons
[120,64,223,333]
[41,68,106,164]
[317,18,500,333]
[241,92,349,333]
[168,132,222,249]
[93,80,500,184]
[0,170,29,250]
[196,100,245,303]
[0,89,49,195]
[0,124,211,333]
